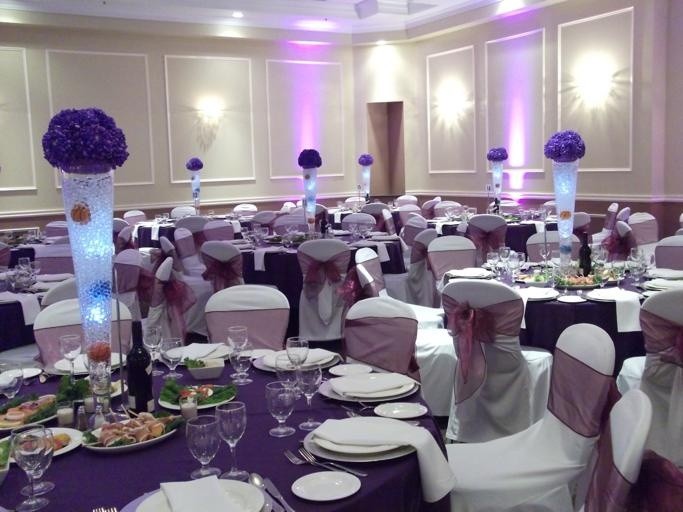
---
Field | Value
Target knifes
[263,477,295,512]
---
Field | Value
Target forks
[282,447,367,477]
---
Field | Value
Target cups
[154,212,169,223]
[178,395,197,422]
[56,401,74,427]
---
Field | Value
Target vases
[57,165,120,405]
[489,159,506,201]
[360,165,371,201]
[300,166,321,239]
[189,170,204,215]
[549,159,583,268]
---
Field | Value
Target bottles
[578,232,592,275]
[125,321,155,417]
[75,406,87,430]
[93,405,103,429]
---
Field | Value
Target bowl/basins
[187,358,225,380]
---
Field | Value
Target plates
[3,367,42,379]
[235,349,339,372]
[134,478,263,512]
[54,351,127,373]
[72,380,128,404]
[442,264,681,302]
[302,427,415,463]
[157,385,236,409]
[165,344,229,361]
[0,394,70,432]
[290,471,361,502]
[81,416,177,454]
[0,427,82,463]
[315,361,428,417]
[0,417,57,432]
[312,417,412,455]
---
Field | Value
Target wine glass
[141,326,164,377]
[185,414,220,479]
[226,325,253,385]
[159,338,184,379]
[59,334,80,384]
[0,258,39,292]
[239,223,269,248]
[215,402,249,482]
[485,248,525,288]
[539,243,549,262]
[0,362,24,399]
[275,336,321,431]
[590,249,656,290]
[9,424,55,512]
[345,220,371,244]
[265,380,296,438]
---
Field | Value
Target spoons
[346,410,419,426]
[247,472,284,511]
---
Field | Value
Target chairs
[1,194,683,512]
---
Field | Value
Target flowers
[37,106,130,175]
[295,145,323,167]
[185,156,202,170]
[487,145,508,162]
[542,127,588,163]
[357,150,372,167]
